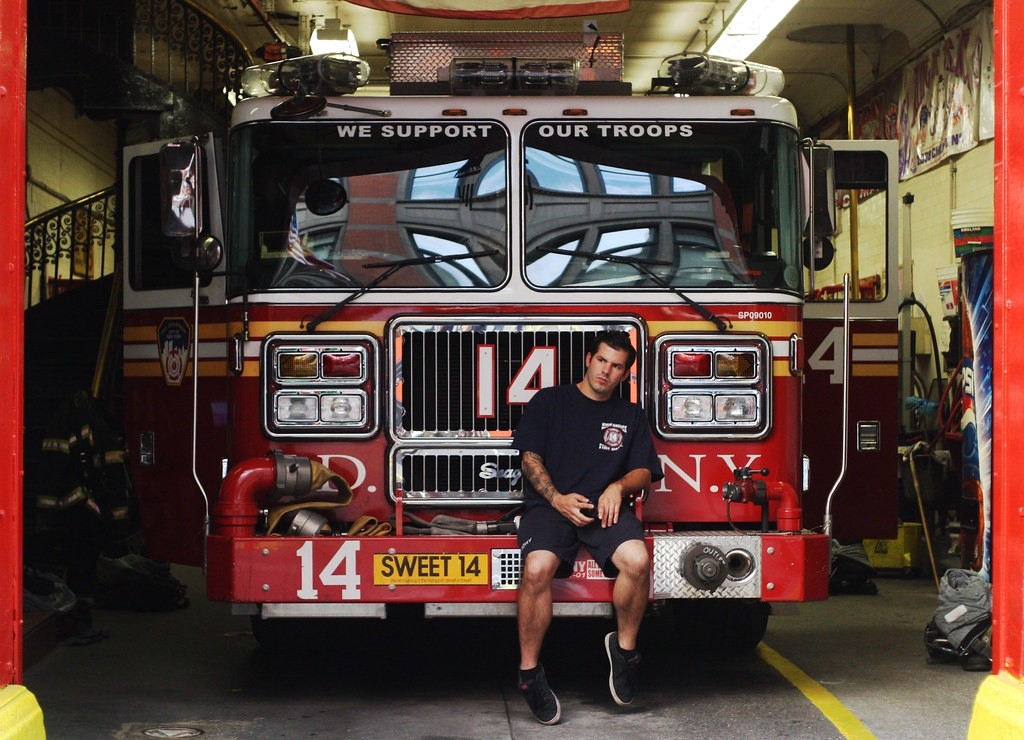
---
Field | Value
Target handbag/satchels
[91,548,188,610]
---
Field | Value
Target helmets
[838,553,879,583]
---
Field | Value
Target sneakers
[604,631,638,706]
[517,661,561,725]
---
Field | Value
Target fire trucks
[115,21,900,655]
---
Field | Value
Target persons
[516,330,665,726]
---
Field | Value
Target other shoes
[962,654,991,670]
